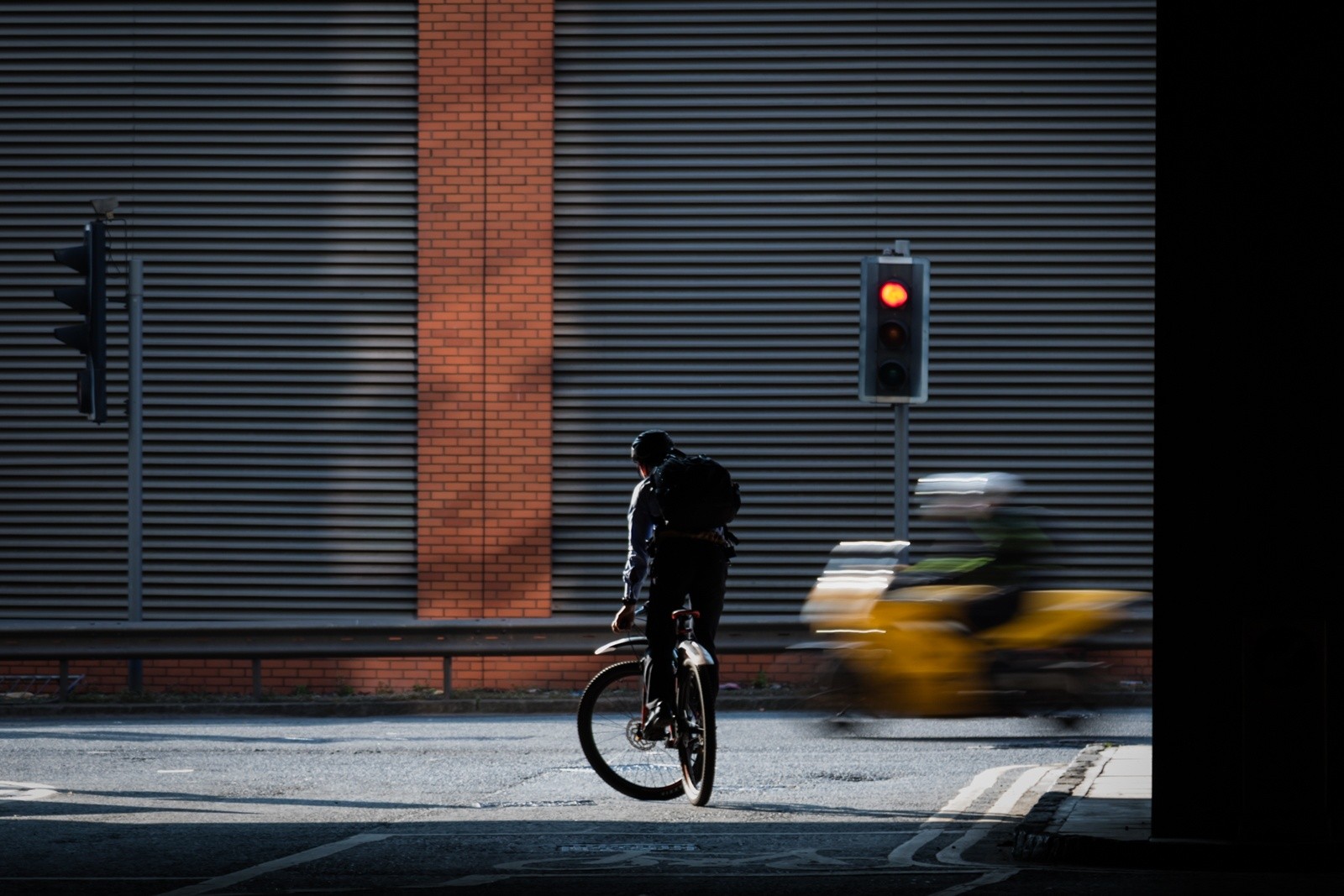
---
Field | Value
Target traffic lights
[52,220,108,426]
[860,252,931,405]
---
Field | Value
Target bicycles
[575,597,720,808]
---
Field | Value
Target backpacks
[651,455,741,545]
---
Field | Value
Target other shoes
[643,705,672,736]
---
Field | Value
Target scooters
[797,538,1153,716]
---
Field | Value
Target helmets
[630,429,674,468]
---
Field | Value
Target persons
[612,431,741,777]
